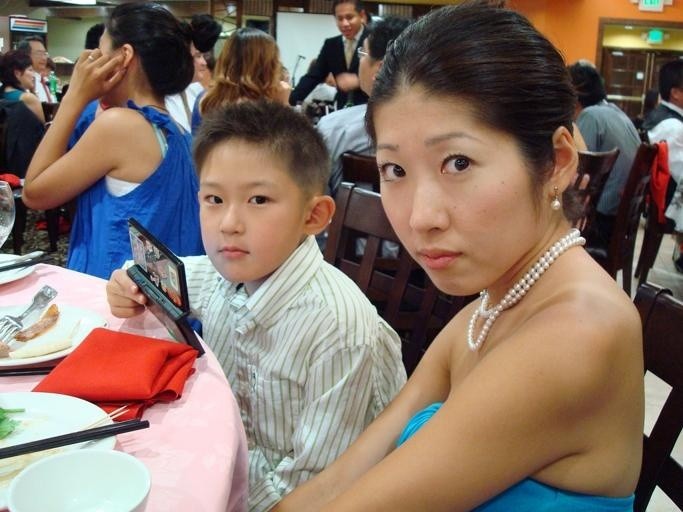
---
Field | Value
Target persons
[0,22,47,179]
[645,58,681,276]
[264,1,644,512]
[566,58,641,245]
[19,35,50,104]
[80,21,105,49]
[105,99,406,511]
[186,1,407,257]
[21,2,204,281]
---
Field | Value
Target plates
[0,390,115,508]
[0,251,34,286]
[0,303,109,366]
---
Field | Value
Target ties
[344,39,355,68]
[41,77,52,104]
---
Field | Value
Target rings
[87,56,94,62]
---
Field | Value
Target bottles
[47,71,56,94]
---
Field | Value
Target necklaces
[467,226,586,350]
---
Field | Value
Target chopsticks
[0,419,151,461]
[0,255,58,272]
[0,364,62,378]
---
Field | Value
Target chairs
[0,98,58,254]
[634,139,683,287]
[632,280,683,511]
[323,181,481,383]
[571,144,620,248]
[585,140,668,299]
[338,150,419,271]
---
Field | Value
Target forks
[0,250,43,266]
[0,284,59,343]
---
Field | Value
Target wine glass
[0,182,15,246]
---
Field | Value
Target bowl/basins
[6,450,151,509]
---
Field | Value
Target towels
[649,139,672,226]
[29,327,200,426]
